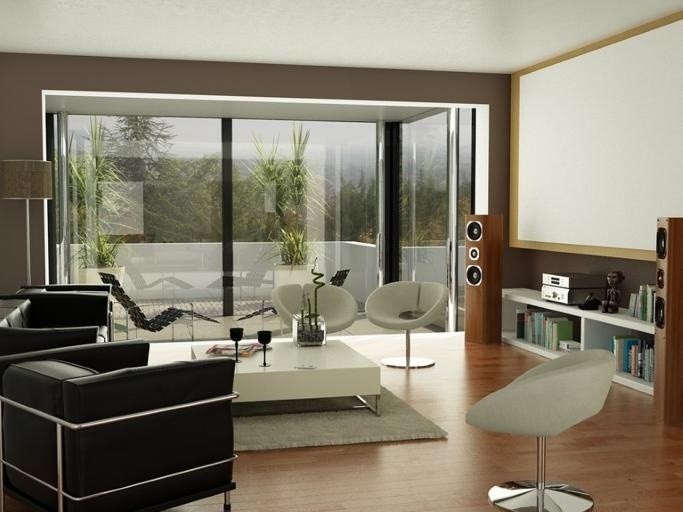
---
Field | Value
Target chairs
[275,279,448,368]
[468,349,618,510]
[97,271,221,342]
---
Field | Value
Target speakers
[465,214,503,345]
[654,217,683,425]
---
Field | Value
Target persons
[601,270,626,313]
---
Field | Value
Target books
[612,335,655,383]
[618,283,656,324]
[523,308,580,353]
[206,342,272,359]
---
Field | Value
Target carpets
[233,384,448,453]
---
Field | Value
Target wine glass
[230,328,243,363]
[258,331,271,367]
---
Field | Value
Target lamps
[1,160,53,285]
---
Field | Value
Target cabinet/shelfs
[502,288,655,396]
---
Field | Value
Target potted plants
[62,114,125,299]
[240,120,319,288]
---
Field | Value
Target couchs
[1,339,240,512]
[0,282,115,356]
